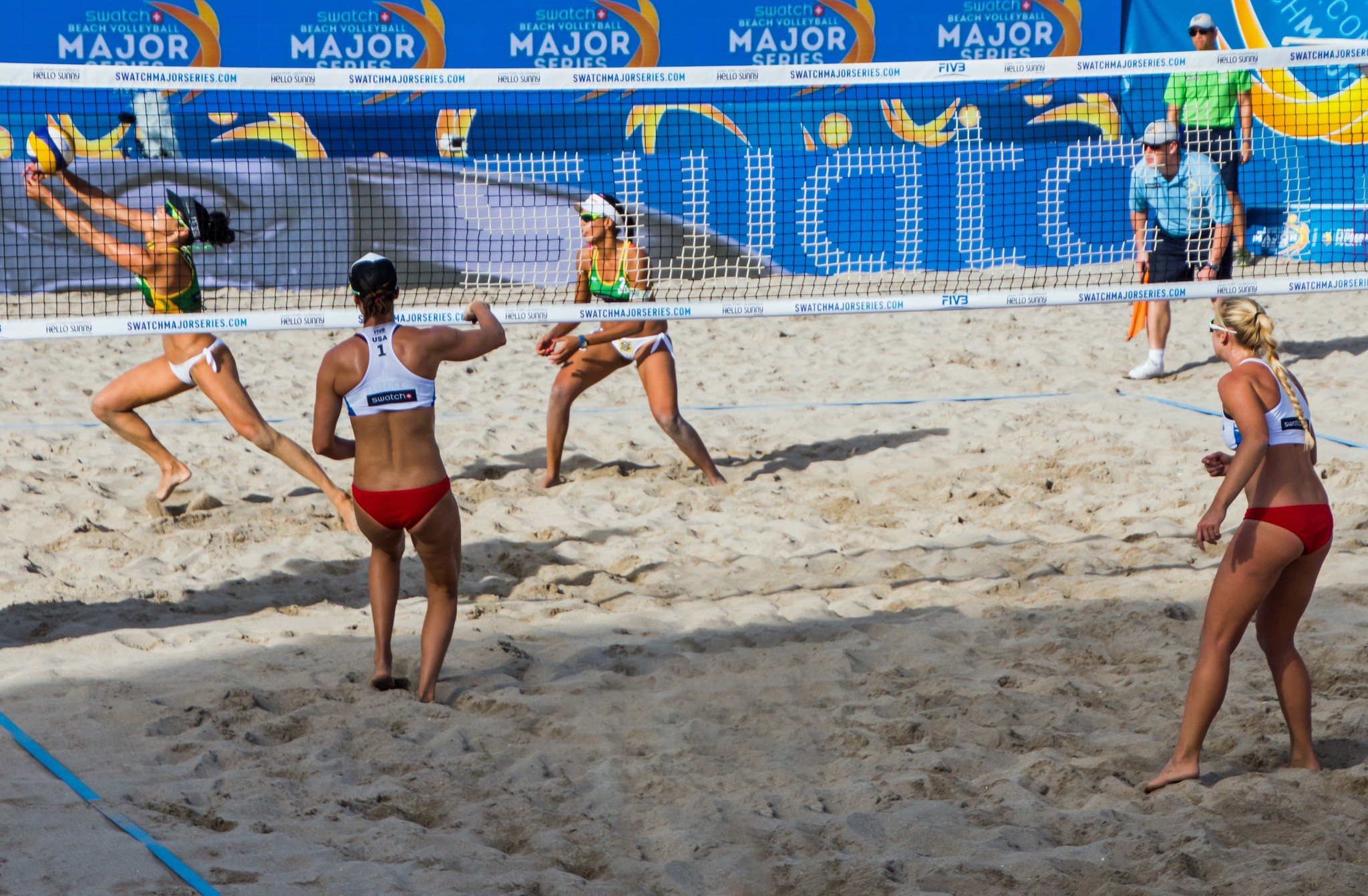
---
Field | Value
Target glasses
[1189,28,1213,36]
[165,200,191,231]
[1209,318,1238,335]
[1141,142,1168,150]
[579,212,608,223]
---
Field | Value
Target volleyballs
[26,125,76,173]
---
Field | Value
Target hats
[573,193,624,226]
[1135,118,1180,144]
[348,252,391,277]
[1187,13,1216,29]
[166,188,201,243]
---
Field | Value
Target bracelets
[1244,139,1252,144]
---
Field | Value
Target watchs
[1206,262,1220,272]
[579,334,588,349]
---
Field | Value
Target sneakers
[1130,356,1165,379]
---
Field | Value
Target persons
[535,192,726,489]
[1163,13,1254,268]
[312,252,507,701]
[1124,119,1234,380]
[21,163,353,532]
[1145,297,1334,794]
